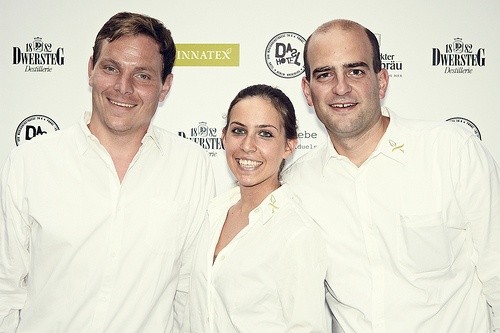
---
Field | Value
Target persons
[179,84,332,333]
[0,11,227,333]
[280,20,500,333]
